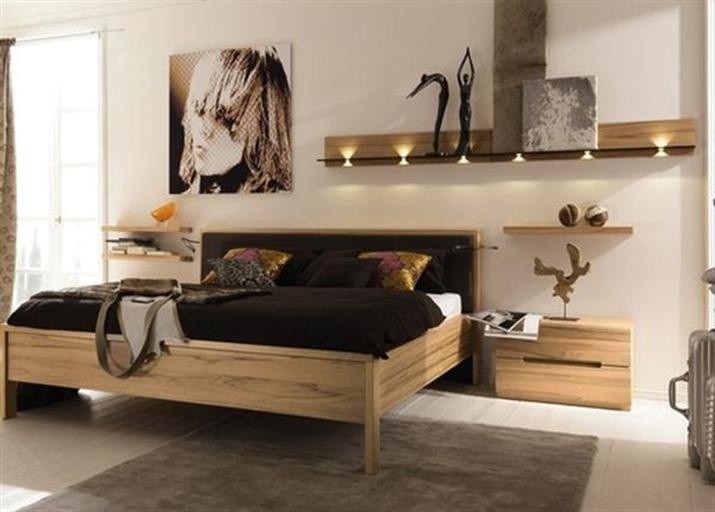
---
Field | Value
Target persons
[457,46,477,158]
[406,74,449,154]
[174,44,293,195]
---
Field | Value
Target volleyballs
[558,203,582,227]
[584,204,609,227]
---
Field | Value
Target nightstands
[492,314,636,409]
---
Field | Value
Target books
[521,75,599,153]
[476,311,545,342]
[467,308,527,334]
[106,235,177,257]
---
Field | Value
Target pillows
[207,247,449,295]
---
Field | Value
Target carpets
[14,413,598,512]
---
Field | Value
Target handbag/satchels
[117,277,182,298]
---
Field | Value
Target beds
[1,227,483,473]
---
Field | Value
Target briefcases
[667,330,715,485]
